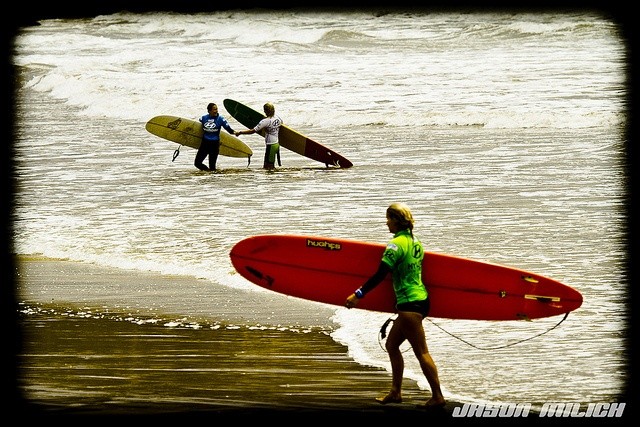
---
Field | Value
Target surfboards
[222,98,354,168]
[230,236,582,353]
[145,115,253,167]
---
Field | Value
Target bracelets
[355,288,363,298]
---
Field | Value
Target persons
[237,103,283,170]
[345,202,446,408]
[194,103,239,172]
